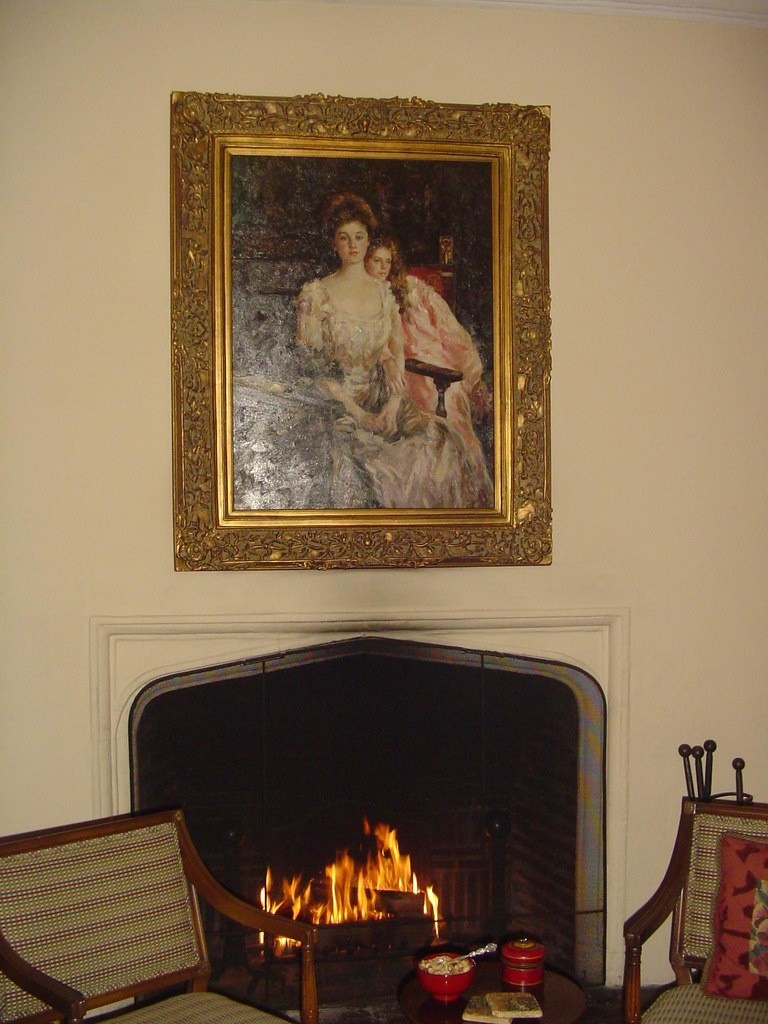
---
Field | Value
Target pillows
[699,831,768,1002]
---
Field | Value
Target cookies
[462,996,513,1024]
[487,993,542,1018]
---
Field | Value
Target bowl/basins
[418,953,476,1001]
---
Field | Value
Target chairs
[0,808,321,1024]
[622,797,768,1024]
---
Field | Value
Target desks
[400,960,588,1024]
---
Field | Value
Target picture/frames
[168,88,556,569]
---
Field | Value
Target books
[462,995,513,1024]
[486,992,543,1018]
